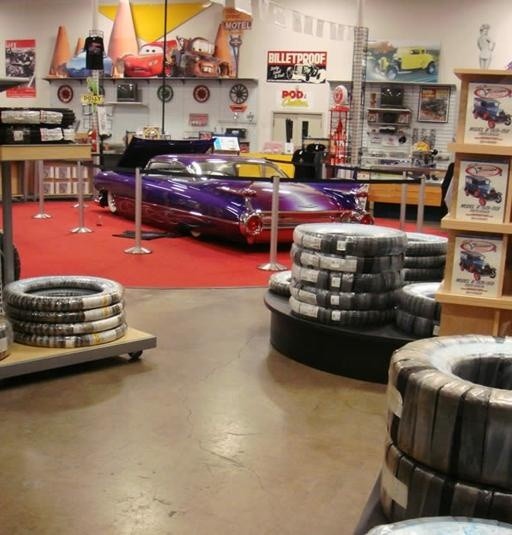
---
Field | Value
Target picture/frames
[416,85,451,124]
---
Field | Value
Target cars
[460,251,496,280]
[472,98,511,127]
[464,175,502,206]
[372,46,436,79]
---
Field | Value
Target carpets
[0,191,447,292]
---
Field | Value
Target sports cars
[94,138,374,239]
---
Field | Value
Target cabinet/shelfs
[366,106,412,128]
[431,66,511,342]
